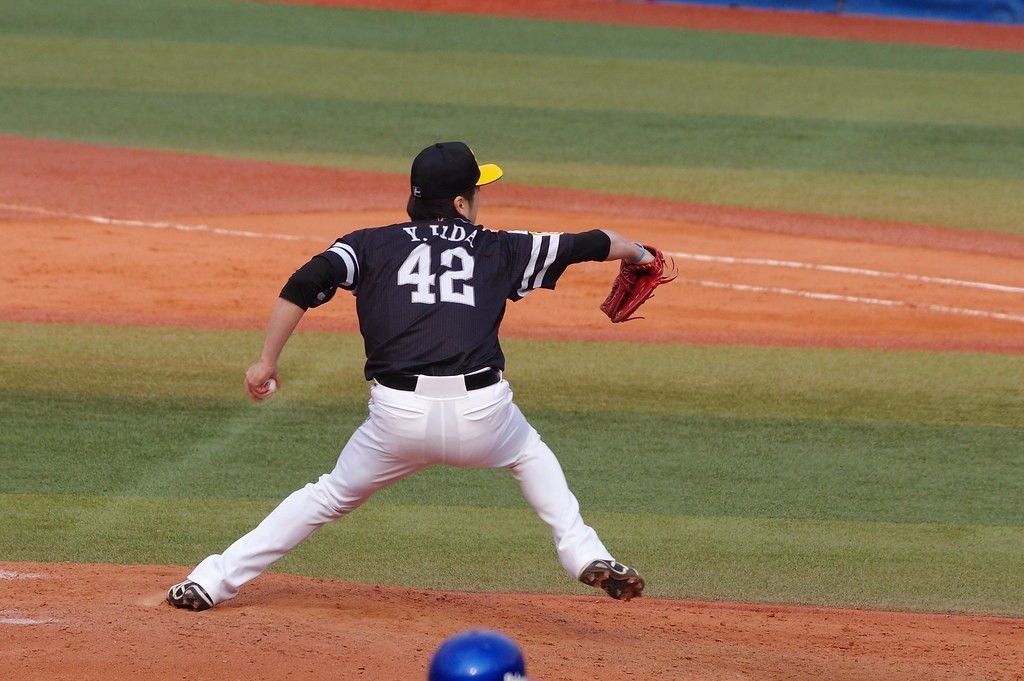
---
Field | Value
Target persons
[167,142,665,609]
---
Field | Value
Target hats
[411,142,503,199]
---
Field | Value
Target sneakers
[578,560,645,601]
[167,580,212,610]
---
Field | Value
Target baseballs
[262,378,277,394]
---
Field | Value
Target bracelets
[628,242,645,262]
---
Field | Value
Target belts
[374,369,501,392]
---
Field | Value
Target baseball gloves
[597,244,680,325]
